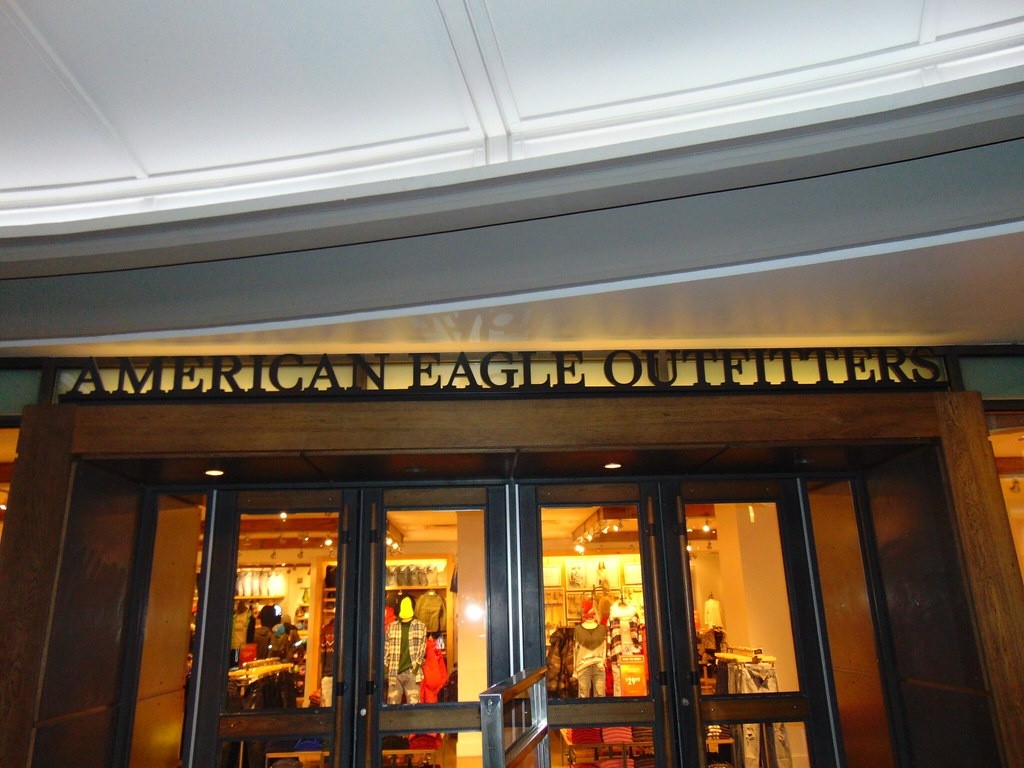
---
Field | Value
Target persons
[569,604,607,698]
[384,595,428,705]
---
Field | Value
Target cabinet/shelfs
[560,730,737,768]
[234,567,290,599]
[304,555,455,707]
[713,646,778,667]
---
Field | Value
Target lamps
[386,537,393,546]
[392,542,399,549]
[686,544,691,552]
[280,512,288,522]
[324,530,332,546]
[686,528,692,533]
[703,520,710,533]
[574,519,624,553]
